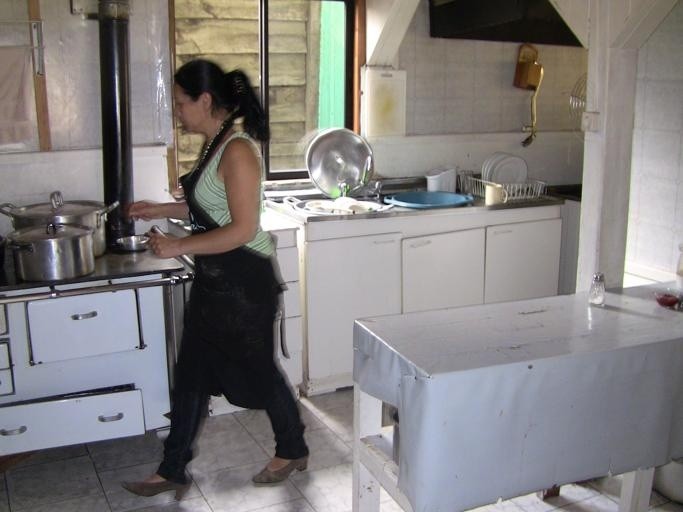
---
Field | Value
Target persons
[120,59,309,499]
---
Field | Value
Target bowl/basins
[652,287,683,310]
[303,195,379,217]
[115,234,152,253]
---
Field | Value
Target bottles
[586,271,607,308]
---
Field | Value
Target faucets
[358,155,373,187]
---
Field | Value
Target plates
[481,150,529,195]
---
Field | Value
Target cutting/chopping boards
[364,68,408,139]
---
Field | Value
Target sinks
[266,197,419,223]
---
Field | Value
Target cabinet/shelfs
[308,219,402,398]
[485,207,561,305]
[0,274,178,457]
[397,212,487,312]
[209,231,300,417]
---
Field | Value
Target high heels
[252,456,308,484]
[119,471,193,500]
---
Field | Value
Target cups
[485,183,508,206]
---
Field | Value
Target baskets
[457,173,547,203]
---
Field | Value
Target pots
[6,221,97,283]
[0,190,121,262]
